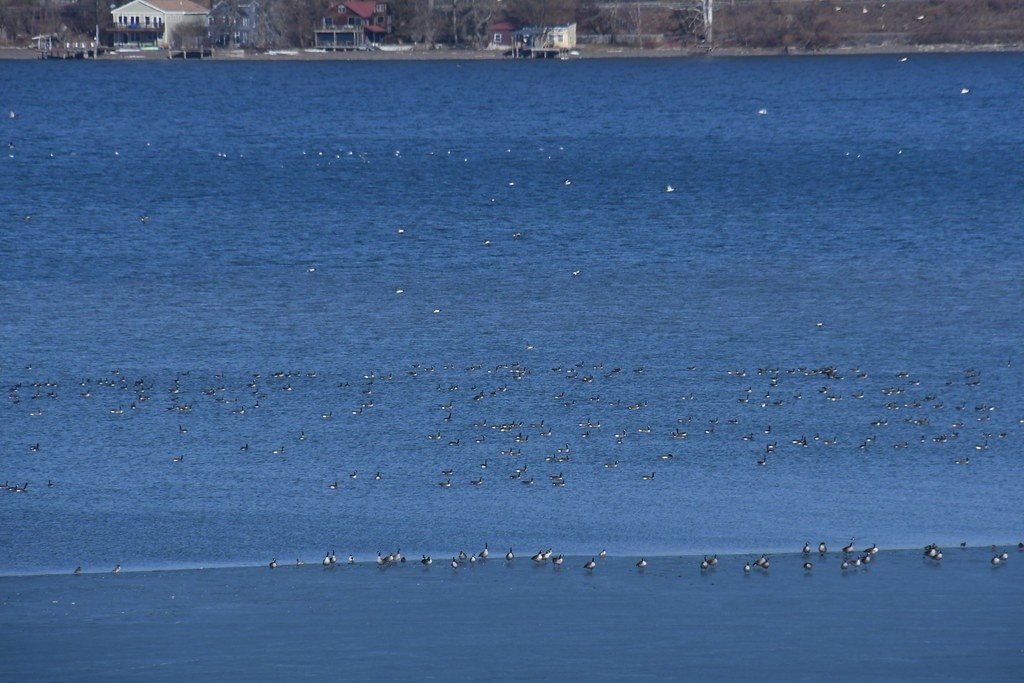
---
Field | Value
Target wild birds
[0,360,1024,496]
[71,541,1024,576]
